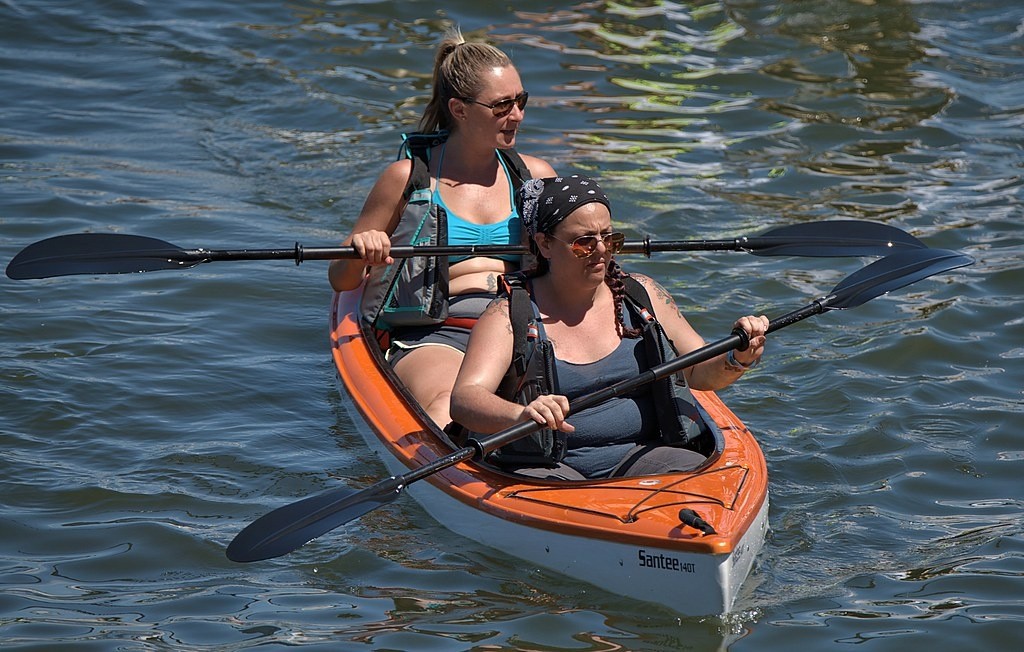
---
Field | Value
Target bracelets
[727,350,760,372]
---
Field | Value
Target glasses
[457,91,528,117]
[546,233,626,259]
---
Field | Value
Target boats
[328,273,769,619]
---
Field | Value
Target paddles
[222,245,976,565]
[7,218,926,282]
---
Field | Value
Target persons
[328,25,558,436]
[449,174,769,480]
